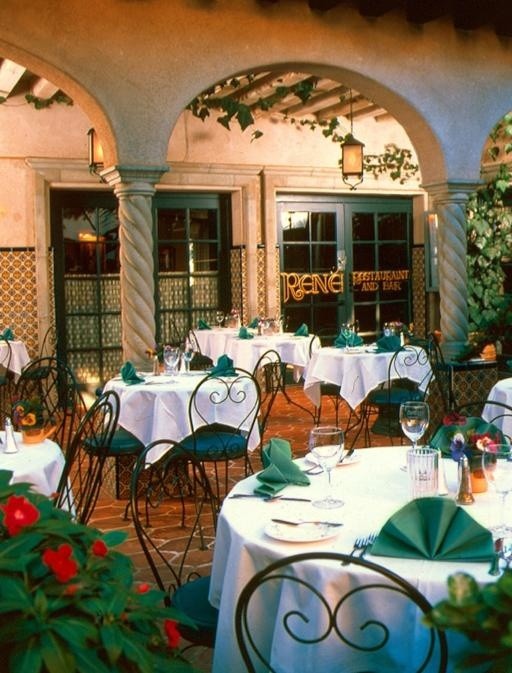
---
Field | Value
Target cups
[383,322,394,337]
[408,447,440,499]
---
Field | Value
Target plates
[342,348,362,354]
[265,513,344,544]
[305,446,360,467]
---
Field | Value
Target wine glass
[215,309,239,330]
[159,343,197,383]
[400,401,430,472]
[481,445,512,535]
[308,426,345,508]
[341,322,356,352]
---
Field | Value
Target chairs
[0,314,511,673]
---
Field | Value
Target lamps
[86,127,107,184]
[337,85,366,190]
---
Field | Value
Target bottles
[437,449,474,505]
[152,355,160,376]
[400,331,404,347]
[2,417,18,454]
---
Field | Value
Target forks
[343,533,377,566]
